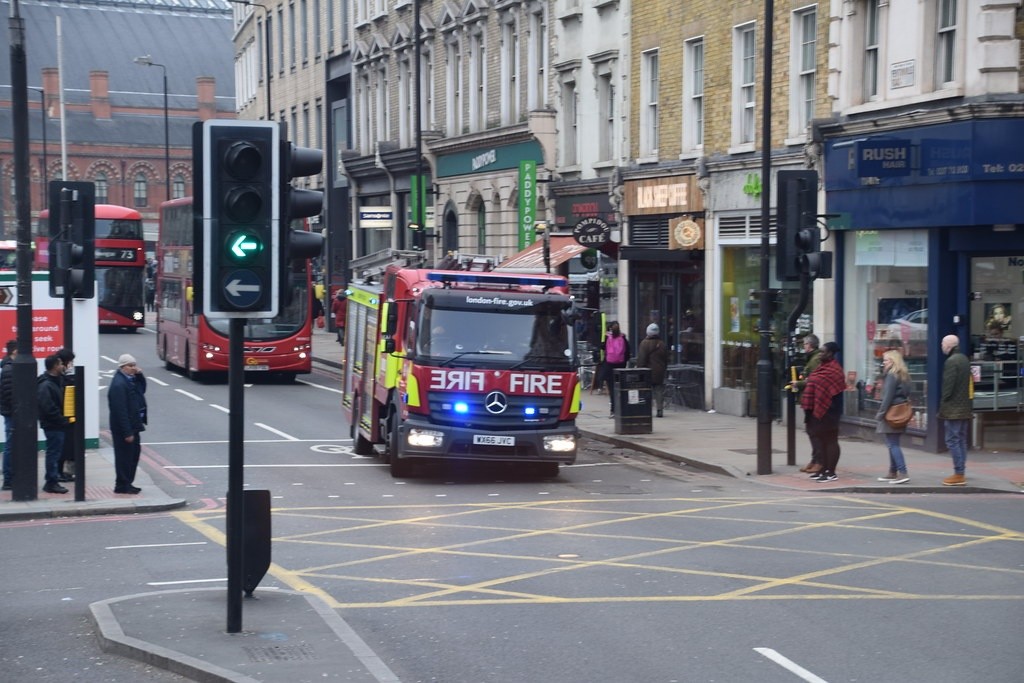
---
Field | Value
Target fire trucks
[339,247,608,482]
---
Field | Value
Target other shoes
[115,484,141,493]
[878,473,898,481]
[807,463,822,473]
[944,475,967,485]
[800,464,814,472]
[3,481,12,489]
[43,481,69,494]
[656,410,663,417]
[57,473,75,482]
[890,473,910,484]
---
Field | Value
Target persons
[410,310,446,352]
[146,287,154,312]
[0,340,17,491]
[332,289,347,346]
[637,324,668,418]
[790,335,844,482]
[602,322,629,417]
[35,349,75,493]
[986,322,1015,359]
[108,355,146,494]
[875,350,912,483]
[937,335,973,486]
[986,304,1011,329]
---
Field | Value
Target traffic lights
[282,137,324,266]
[202,118,281,319]
[794,227,821,276]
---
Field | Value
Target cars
[873,307,928,367]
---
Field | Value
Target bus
[32,204,147,333]
[152,194,313,385]
[0,240,17,271]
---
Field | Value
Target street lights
[27,86,48,209]
[70,267,85,294]
[227,0,272,122]
[133,54,171,201]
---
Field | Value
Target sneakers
[817,472,839,483]
[809,468,829,479]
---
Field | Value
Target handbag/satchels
[885,402,914,427]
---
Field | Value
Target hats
[646,323,659,337]
[118,354,136,367]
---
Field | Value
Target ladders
[347,249,428,280]
[437,250,503,273]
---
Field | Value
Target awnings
[496,234,587,274]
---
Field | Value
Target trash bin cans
[613,368,653,435]
